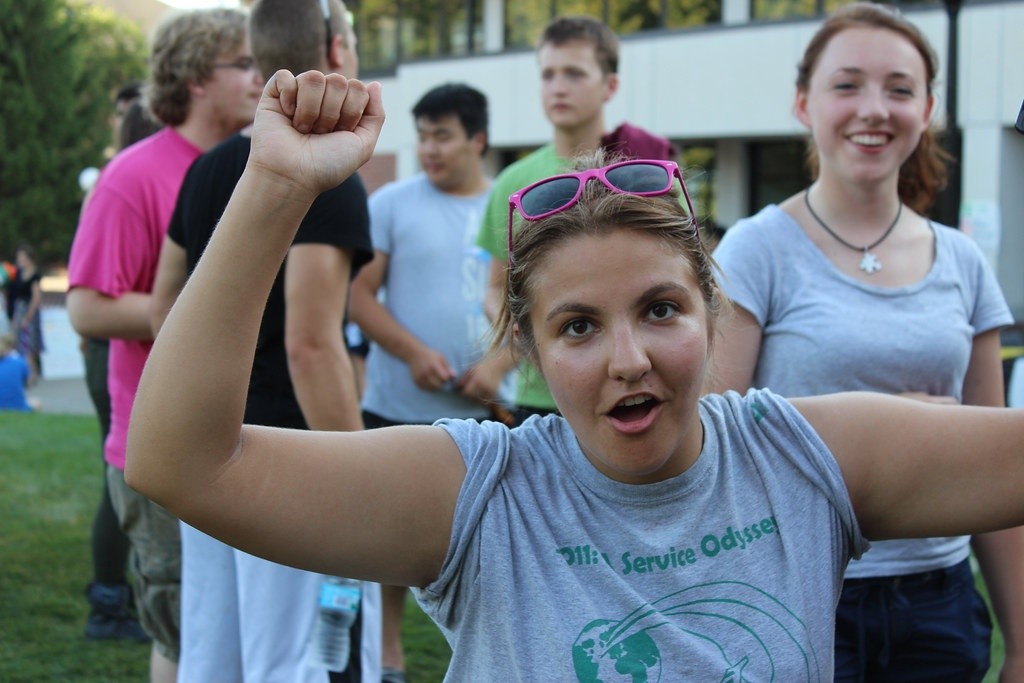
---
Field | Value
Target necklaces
[802,184,904,275]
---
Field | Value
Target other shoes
[380,667,406,682]
[83,582,128,638]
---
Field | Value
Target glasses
[507,161,699,253]
[208,57,256,70]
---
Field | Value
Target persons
[121,67,1024,682]
[348,81,529,682]
[64,0,373,682]
[1,246,47,413]
[706,2,1024,683]
[481,0,697,431]
